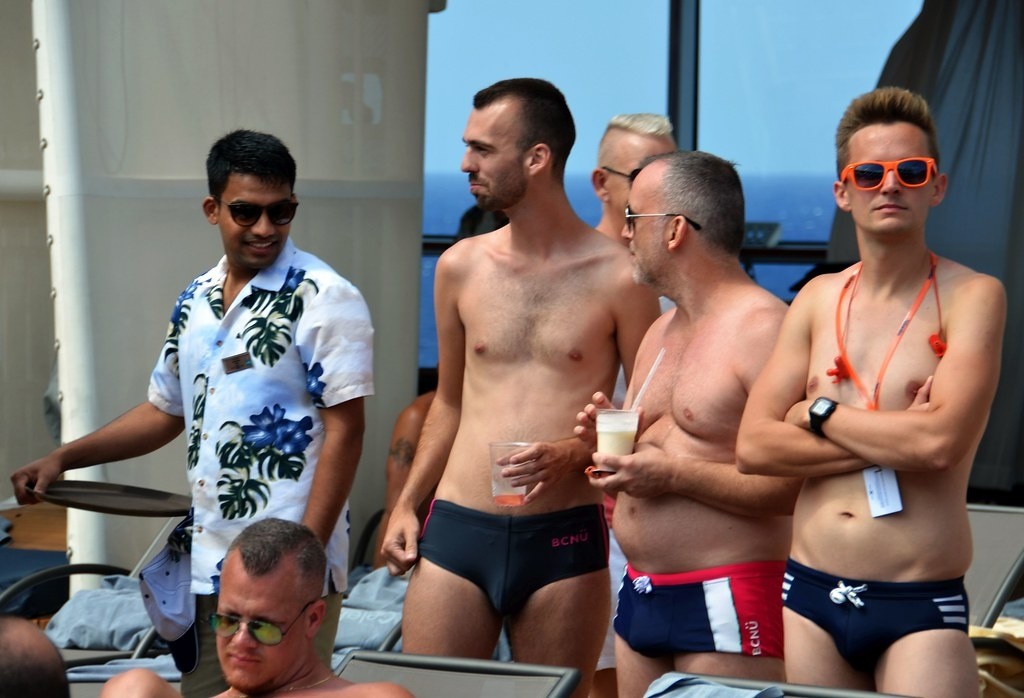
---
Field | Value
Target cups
[593,408,639,472]
[491,443,532,507]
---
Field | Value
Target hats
[141,539,200,675]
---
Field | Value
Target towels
[42,575,168,652]
[64,565,416,682]
[645,666,782,698]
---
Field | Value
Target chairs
[332,550,1024,698]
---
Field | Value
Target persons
[0,617,70,698]
[588,110,681,414]
[380,82,664,673]
[104,517,415,698]
[10,127,378,698]
[377,363,441,570]
[732,87,1008,698]
[572,149,802,698]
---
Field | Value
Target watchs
[808,397,839,438]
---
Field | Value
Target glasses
[209,604,312,645]
[625,205,700,237]
[601,165,641,187]
[840,157,938,191]
[227,200,299,227]
